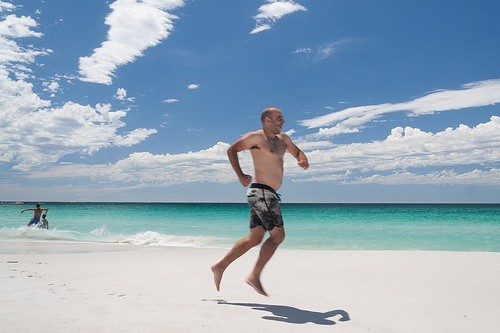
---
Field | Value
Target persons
[21,203,48,227]
[40,214,48,230]
[210,108,309,297]
